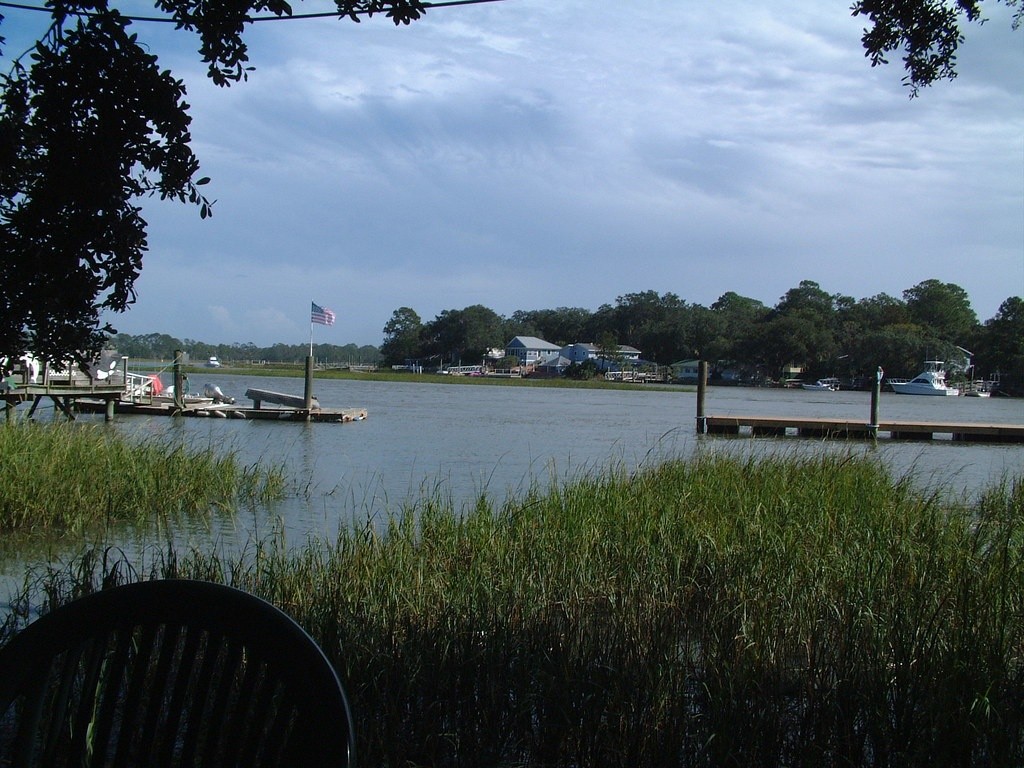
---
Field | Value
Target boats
[885,358,960,397]
[966,390,991,398]
[802,382,835,391]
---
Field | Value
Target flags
[311,302,336,326]
